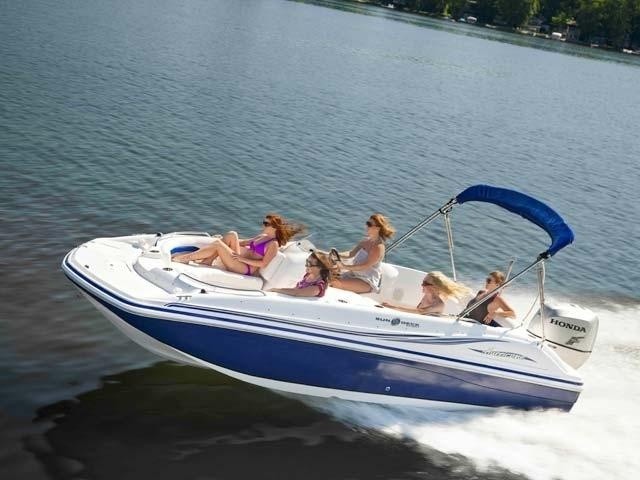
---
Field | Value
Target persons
[267,250,339,297]
[382,270,472,315]
[171,213,306,274]
[328,213,395,294]
[458,270,517,324]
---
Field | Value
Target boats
[59,187,599,411]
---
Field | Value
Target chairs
[172,251,285,291]
[357,263,398,304]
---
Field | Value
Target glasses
[263,221,272,226]
[487,278,495,284]
[366,222,376,226]
[423,280,433,286]
[306,259,320,267]
